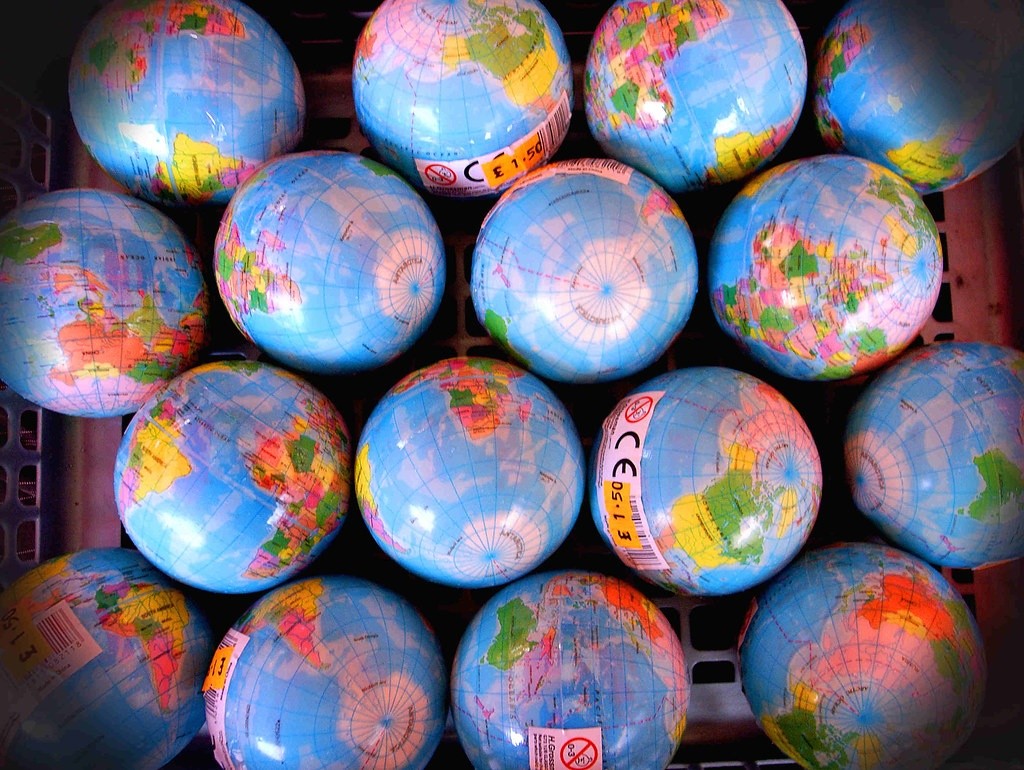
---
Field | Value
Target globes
[733,545,989,770]
[703,156,943,380]
[588,367,824,602]
[450,575,693,770]
[351,353,587,591]
[111,360,354,596]
[470,160,701,387]
[583,0,807,190]
[211,151,448,375]
[811,1,1024,198]
[352,2,575,199]
[843,344,1024,578]
[66,1,309,208]
[199,575,449,770]
[1,548,217,770]
[0,187,208,420]
